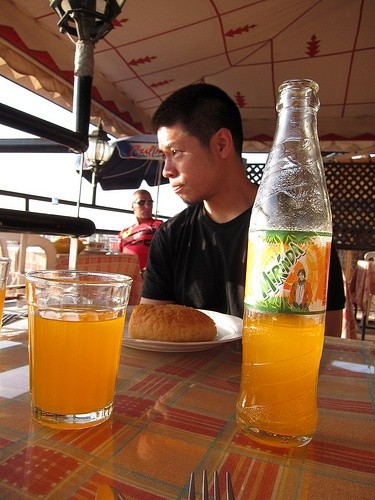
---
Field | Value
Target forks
[2,312,17,327]
[187,469,235,500]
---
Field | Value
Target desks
[0,303,375,500]
[0,246,144,306]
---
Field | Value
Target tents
[0,0,374,153]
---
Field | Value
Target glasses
[134,199,154,205]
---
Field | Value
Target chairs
[0,207,375,340]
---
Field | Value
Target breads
[129,303,218,342]
[52,237,86,253]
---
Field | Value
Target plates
[121,304,244,353]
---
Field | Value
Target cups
[24,269,133,430]
[109,238,122,255]
[0,256,12,328]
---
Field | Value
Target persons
[140,83,347,338]
[113,190,165,271]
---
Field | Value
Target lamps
[84,118,113,205]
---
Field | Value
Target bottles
[235,78,334,453]
